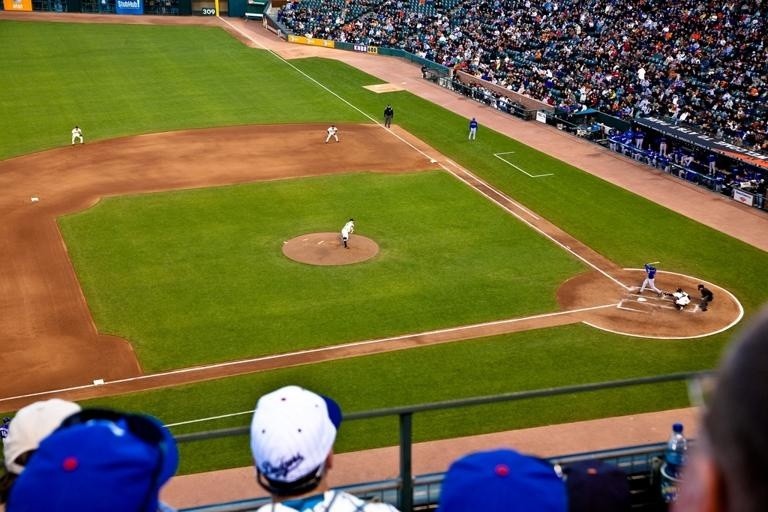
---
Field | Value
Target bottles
[665,422,686,475]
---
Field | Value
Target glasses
[64,407,168,448]
[255,467,322,498]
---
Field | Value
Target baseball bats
[644,262,661,266]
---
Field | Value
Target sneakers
[699,304,708,310]
[680,306,684,311]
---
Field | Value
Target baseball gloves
[662,291,669,296]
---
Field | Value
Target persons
[71,126,84,146]
[276,1,487,106]
[384,105,393,128]
[663,287,691,310]
[637,263,663,296]
[696,284,714,311]
[325,125,340,144]
[340,218,355,249]
[468,118,478,140]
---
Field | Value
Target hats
[438,447,572,510]
[249,383,343,482]
[563,458,632,511]
[3,397,83,476]
[6,407,179,511]
